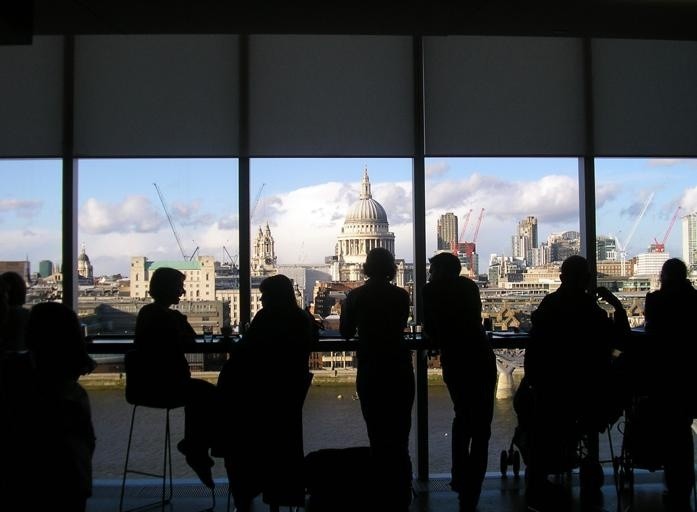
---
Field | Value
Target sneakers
[177,439,216,489]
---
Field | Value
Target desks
[88,323,653,355]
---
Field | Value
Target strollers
[613,394,697,512]
[499,379,604,508]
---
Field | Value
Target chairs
[117,344,200,510]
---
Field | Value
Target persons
[211,274,317,510]
[523,255,635,436]
[636,257,694,501]
[420,250,497,508]
[337,247,417,511]
[0,300,96,511]
[0,268,30,355]
[131,266,219,489]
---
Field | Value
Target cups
[202,325,213,342]
